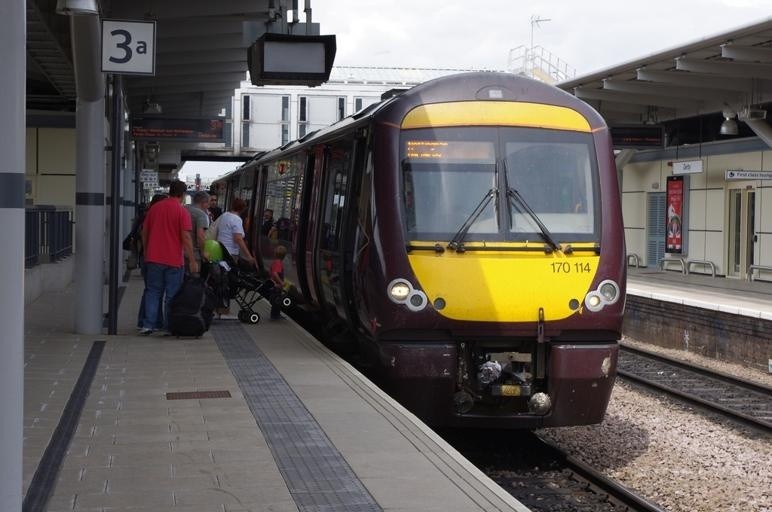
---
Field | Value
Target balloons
[203,239,225,266]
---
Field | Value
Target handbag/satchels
[123,230,135,250]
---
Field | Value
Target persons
[138,179,200,337]
[184,190,212,277]
[240,200,339,253]
[265,245,289,320]
[211,198,257,320]
[208,193,223,222]
[136,193,169,332]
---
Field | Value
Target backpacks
[166,271,219,338]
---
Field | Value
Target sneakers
[220,307,238,320]
[137,326,153,336]
[157,330,172,336]
[212,307,220,320]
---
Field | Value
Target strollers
[207,240,295,325]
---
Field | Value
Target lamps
[718,106,740,135]
[144,139,161,162]
[643,105,657,125]
[55,1,98,17]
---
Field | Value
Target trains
[209,71,630,429]
